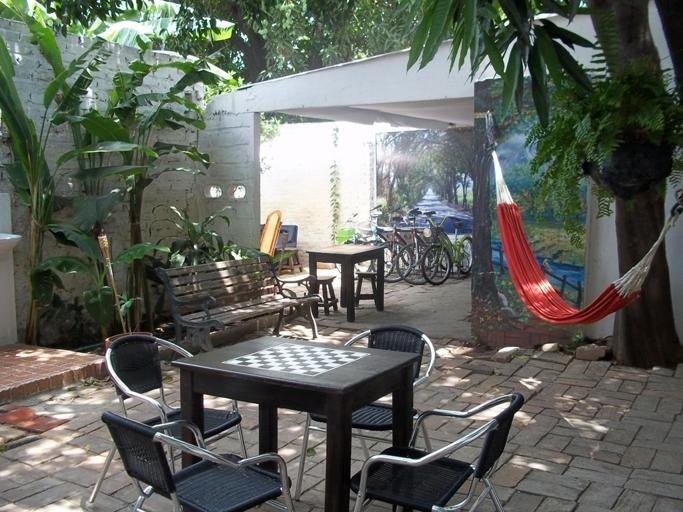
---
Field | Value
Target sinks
[0,233,23,252]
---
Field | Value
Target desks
[305,245,389,322]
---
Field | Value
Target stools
[355,272,378,307]
[277,248,303,273]
[317,275,338,317]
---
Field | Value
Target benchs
[154,253,323,365]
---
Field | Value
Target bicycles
[334,203,471,286]
